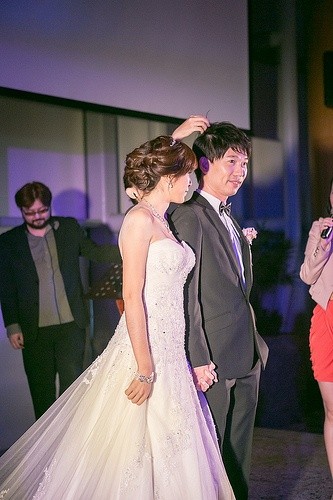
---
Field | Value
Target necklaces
[142,197,173,236]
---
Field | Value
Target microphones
[321,209,333,239]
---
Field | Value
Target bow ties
[219,202,231,216]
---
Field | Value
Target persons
[166,111,270,500]
[0,117,236,500]
[299,184,333,479]
[122,114,211,205]
[0,181,122,421]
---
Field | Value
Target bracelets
[136,372,156,383]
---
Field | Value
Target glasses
[22,206,48,216]
